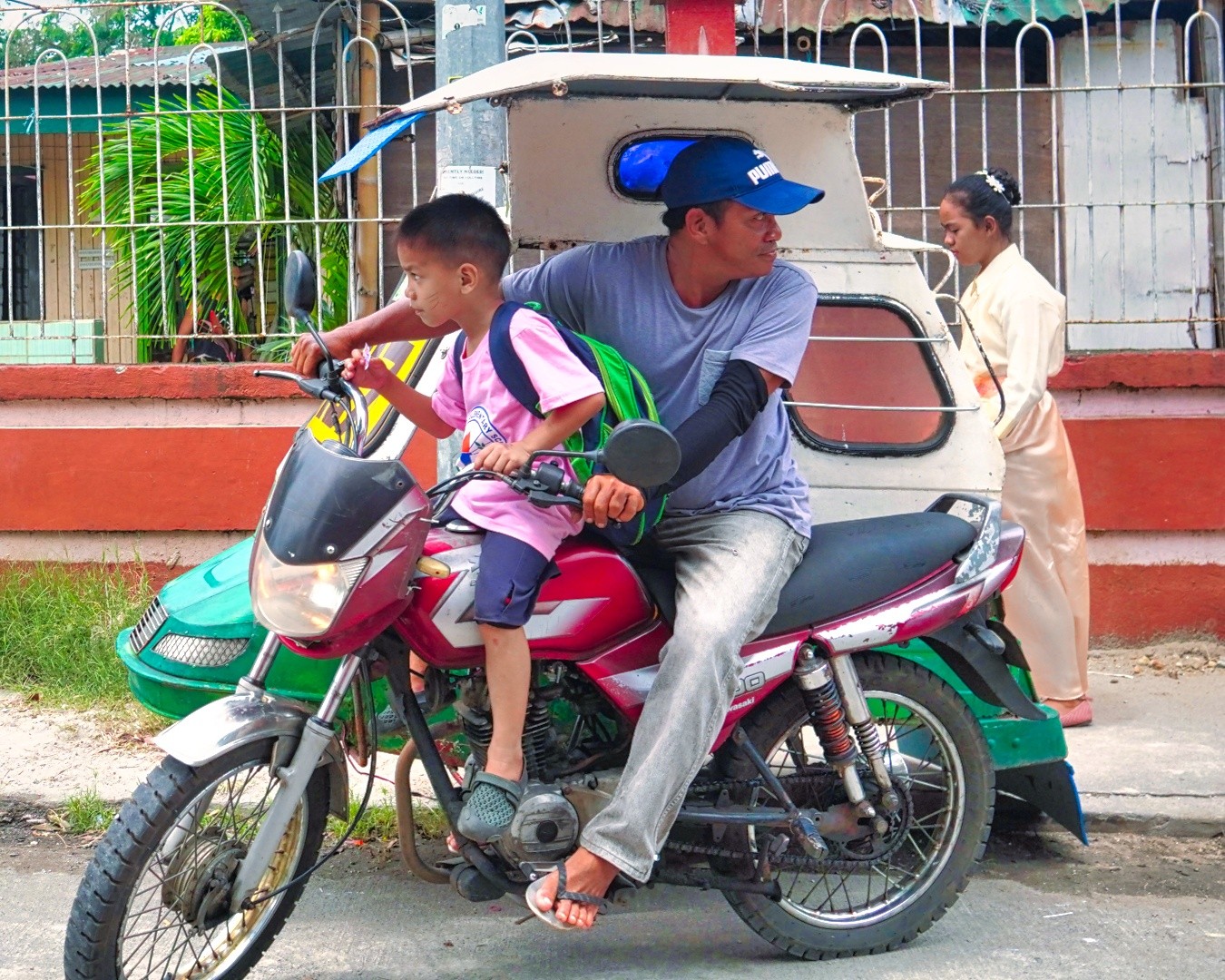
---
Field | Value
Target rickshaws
[55,48,1092,980]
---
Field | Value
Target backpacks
[452,304,668,554]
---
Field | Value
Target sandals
[367,691,430,737]
[456,753,529,844]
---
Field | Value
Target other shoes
[1057,697,1093,728]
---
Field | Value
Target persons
[334,193,606,845]
[290,136,819,936]
[171,232,256,363]
[939,166,1095,732]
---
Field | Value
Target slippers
[526,859,603,931]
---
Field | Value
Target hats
[660,136,825,215]
